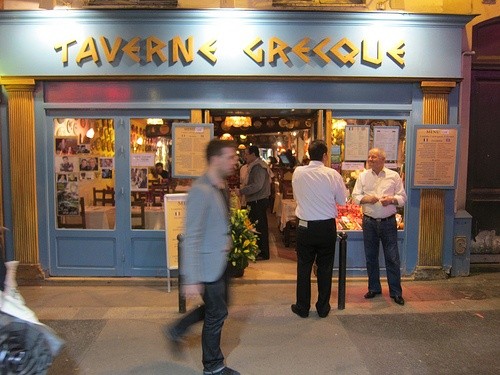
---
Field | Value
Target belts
[367,214,394,222]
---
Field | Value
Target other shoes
[291,303,308,318]
[160,322,187,355]
[391,295,406,305]
[203,364,240,375]
[364,289,383,300]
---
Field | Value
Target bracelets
[391,198,395,204]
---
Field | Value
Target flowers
[229,208,258,263]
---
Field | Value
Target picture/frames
[57,156,113,183]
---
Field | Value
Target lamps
[86,127,94,144]
[40,0,73,12]
[135,137,143,153]
[224,117,252,128]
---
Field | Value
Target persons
[156,163,168,178]
[57,138,147,189]
[241,146,270,260]
[163,140,241,375]
[351,148,406,305]
[291,139,347,318]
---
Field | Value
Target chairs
[57,178,169,229]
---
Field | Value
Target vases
[227,258,250,279]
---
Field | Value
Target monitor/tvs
[277,155,290,166]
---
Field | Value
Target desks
[132,205,165,229]
[130,187,170,206]
[62,206,115,229]
